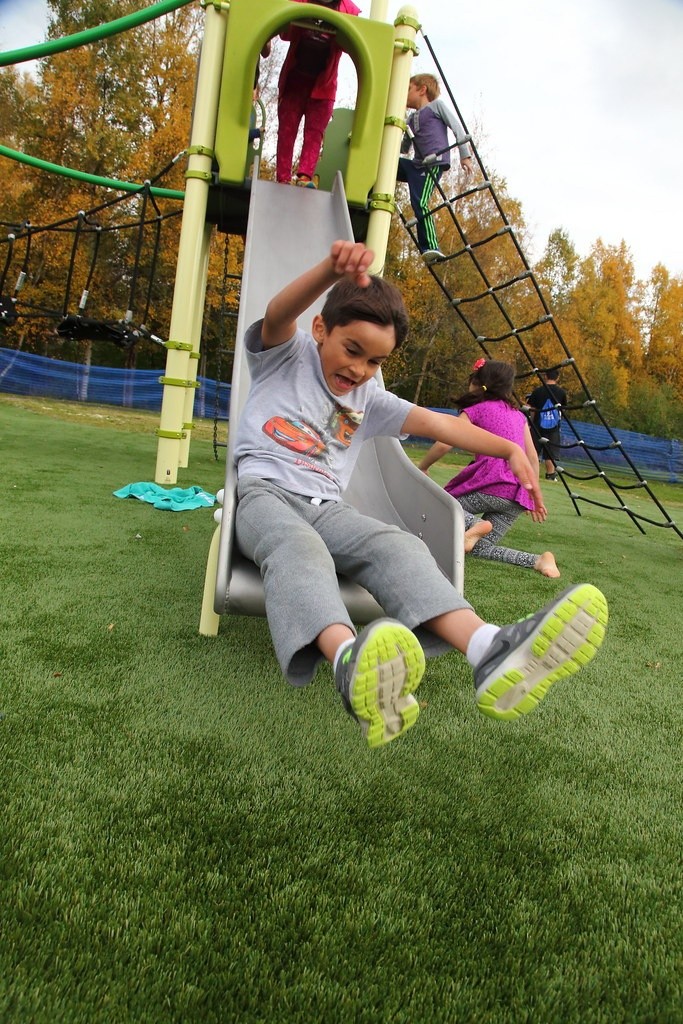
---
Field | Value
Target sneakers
[473,583,609,721]
[335,616,426,748]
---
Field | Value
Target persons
[396,74,472,265]
[211,40,271,171]
[277,0,362,188]
[233,240,608,747]
[526,369,567,481]
[418,358,560,578]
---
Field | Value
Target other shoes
[545,473,558,482]
[279,180,291,184]
[421,247,446,261]
[296,177,317,189]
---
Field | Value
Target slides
[212,157,467,630]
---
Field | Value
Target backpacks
[537,386,562,429]
